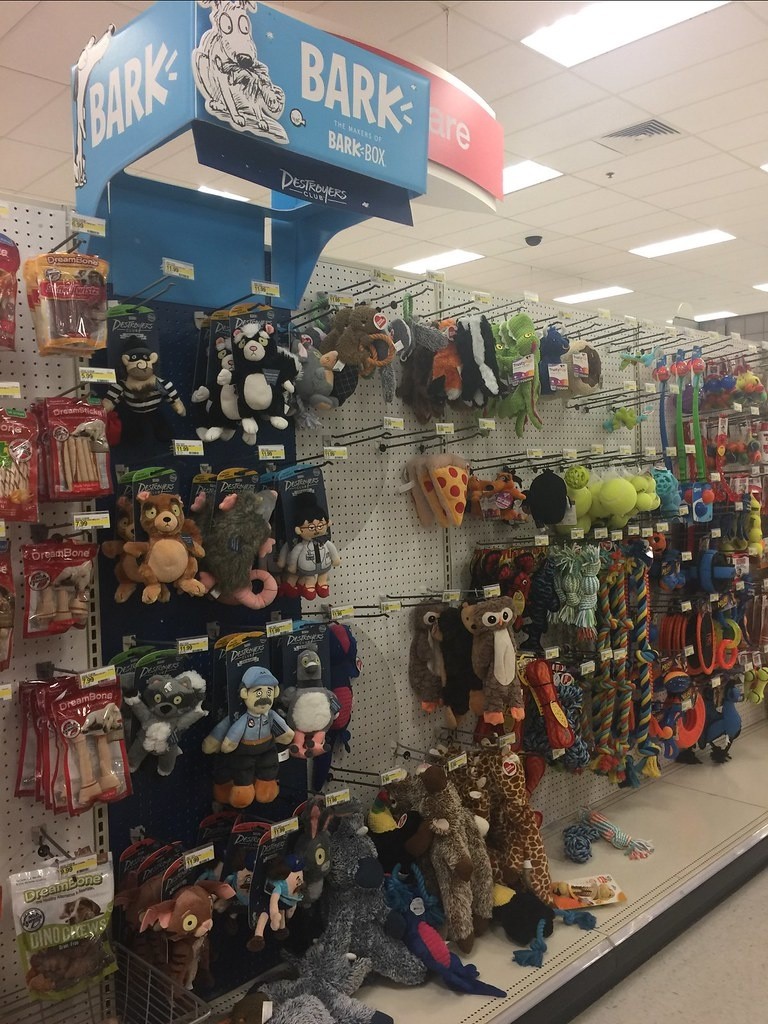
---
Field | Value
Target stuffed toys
[393,312,768,797]
[94,317,597,1023]
